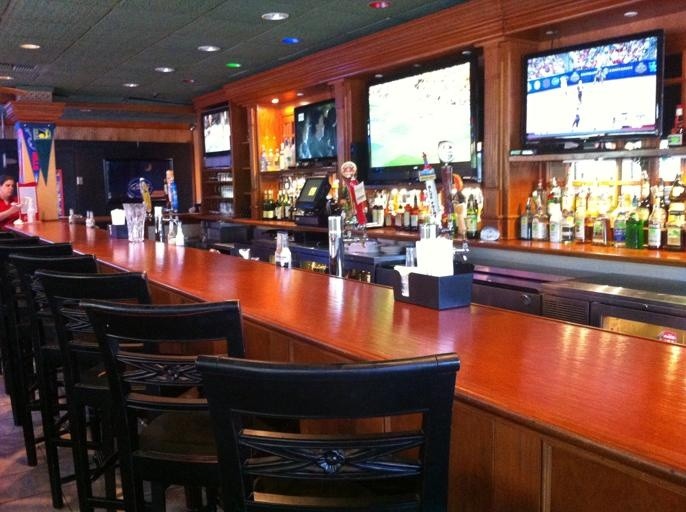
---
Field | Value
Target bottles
[154,206,185,245]
[274,231,292,269]
[668,107,685,147]
[68,208,76,225]
[518,174,686,253]
[86,210,95,228]
[369,187,482,241]
[260,132,298,224]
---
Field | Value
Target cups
[154,245,187,275]
[217,172,233,215]
[122,203,147,242]
[128,245,146,270]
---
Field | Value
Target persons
[126,158,178,211]
[0,174,20,232]
[526,36,657,130]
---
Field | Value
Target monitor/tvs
[295,178,327,209]
[200,105,231,155]
[362,47,485,184]
[294,98,337,167]
[520,29,665,145]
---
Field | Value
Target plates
[348,240,403,258]
